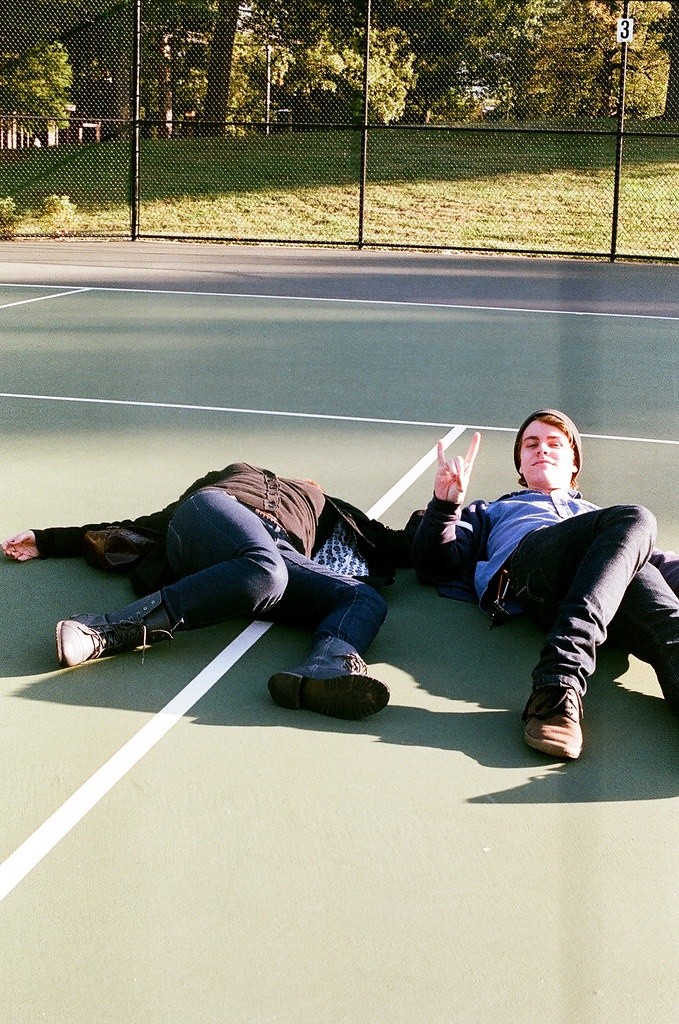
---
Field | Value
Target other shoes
[521,687,583,759]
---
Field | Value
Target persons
[0,459,429,721]
[412,407,679,760]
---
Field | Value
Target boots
[267,636,391,720]
[55,591,174,667]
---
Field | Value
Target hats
[513,409,583,480]
